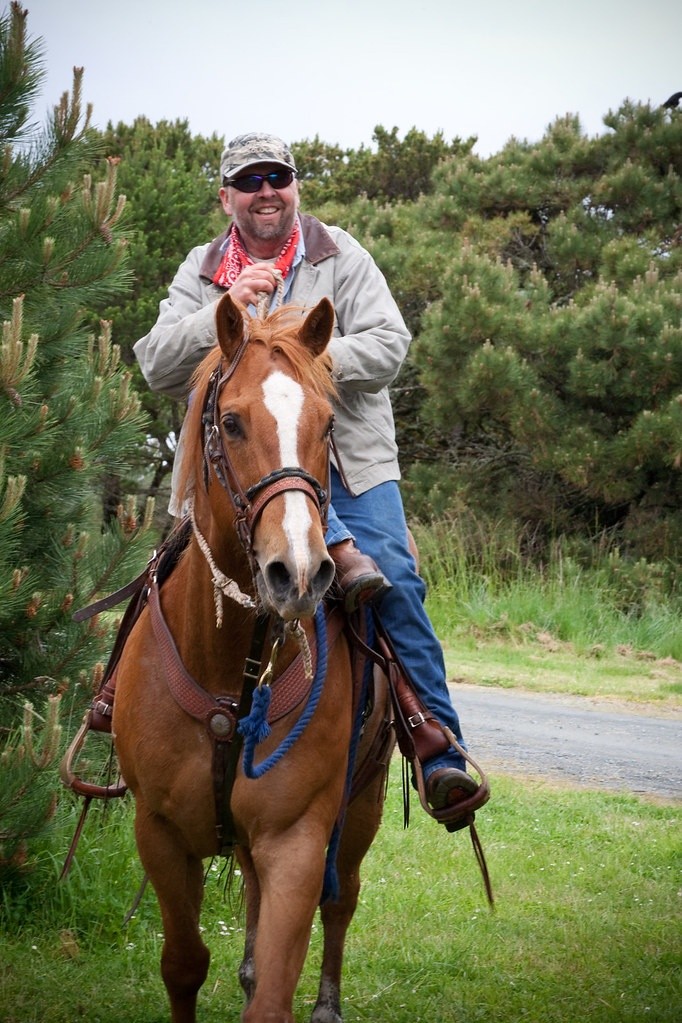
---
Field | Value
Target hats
[220,131,298,188]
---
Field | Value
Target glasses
[225,169,296,193]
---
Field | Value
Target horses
[111,292,396,1022]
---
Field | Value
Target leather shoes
[429,767,477,834]
[330,546,392,613]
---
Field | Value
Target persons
[131,133,478,834]
